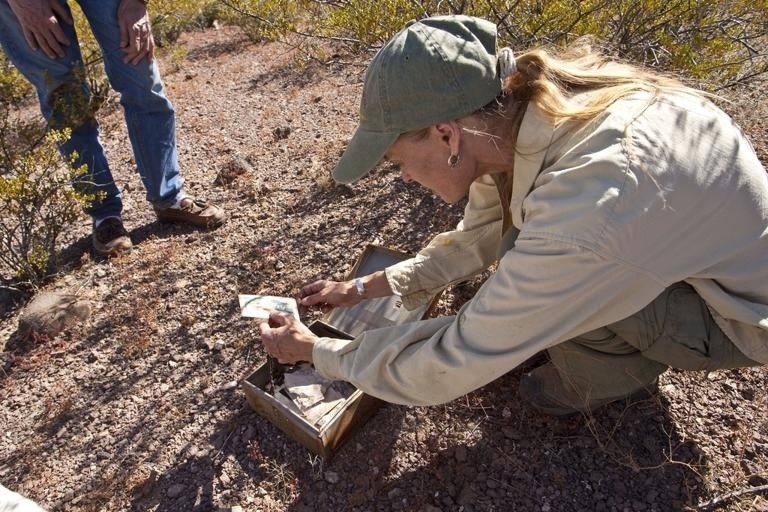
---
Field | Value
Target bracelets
[356,277,368,300]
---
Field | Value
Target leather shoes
[93,213,133,251]
[155,188,225,228]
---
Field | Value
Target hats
[331,14,501,185]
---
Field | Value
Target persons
[0,0,228,253]
[260,13,768,415]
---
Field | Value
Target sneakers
[520,350,668,416]
